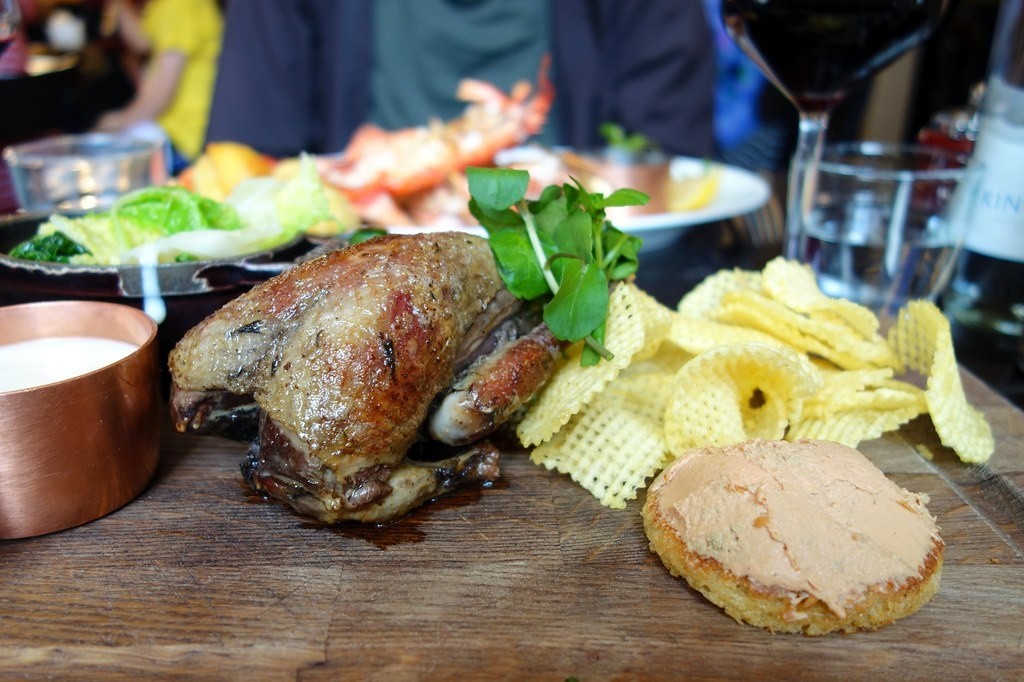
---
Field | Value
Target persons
[0,1,227,215]
[203,0,713,314]
[704,1,1000,248]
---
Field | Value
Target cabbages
[40,154,324,271]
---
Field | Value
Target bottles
[951,0,1024,335]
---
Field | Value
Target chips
[507,256,994,511]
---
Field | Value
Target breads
[641,436,943,635]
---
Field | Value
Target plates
[2,201,324,323]
[313,146,771,256]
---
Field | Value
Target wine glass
[718,0,953,274]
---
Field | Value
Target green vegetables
[465,166,651,364]
[8,230,90,265]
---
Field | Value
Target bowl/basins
[0,298,161,542]
[3,133,157,214]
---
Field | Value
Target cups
[789,143,985,318]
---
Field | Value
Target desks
[0,312,1024,682]
